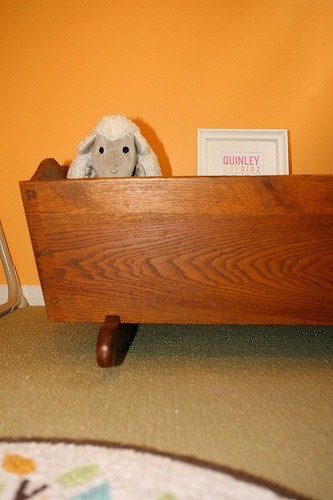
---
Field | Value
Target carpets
[0,305,333,500]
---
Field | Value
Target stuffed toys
[64,112,166,180]
